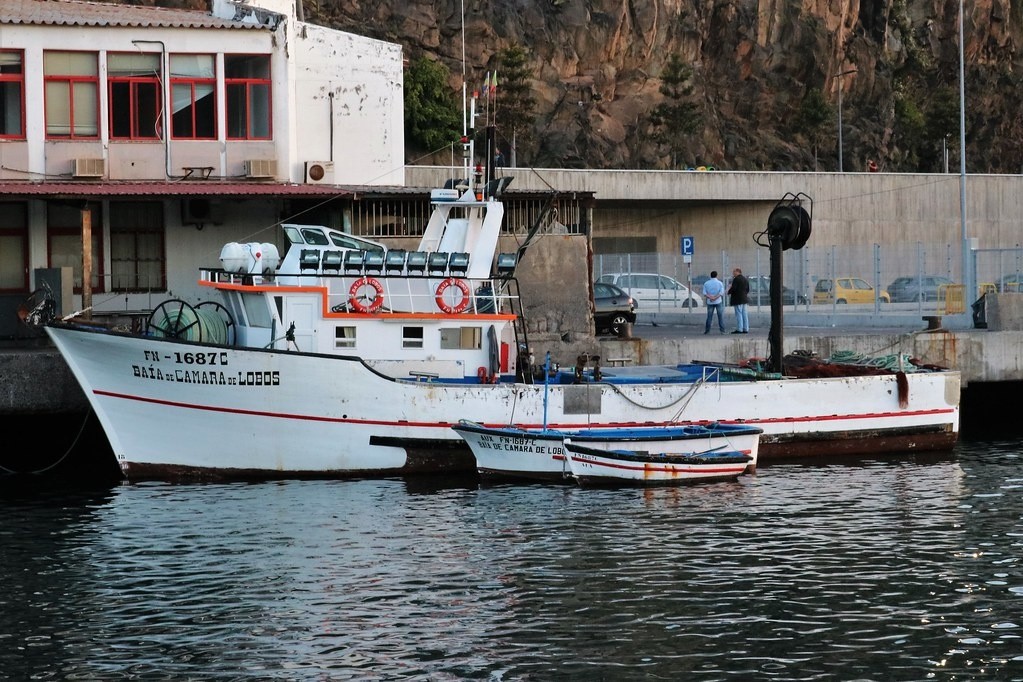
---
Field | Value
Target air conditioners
[70,157,106,177]
[243,158,278,178]
[305,162,335,185]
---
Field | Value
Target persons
[727,267,750,334]
[702,271,727,335]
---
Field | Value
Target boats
[447,418,776,475]
[17,0,964,487]
[559,438,756,486]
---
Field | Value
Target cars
[728,277,811,305]
[967,274,1023,330]
[814,278,891,304]
[591,283,636,336]
[887,277,957,304]
[594,272,703,327]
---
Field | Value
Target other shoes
[731,330,743,334]
[743,330,749,333]
[721,330,729,335]
[705,331,712,335]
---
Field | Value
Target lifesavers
[435,278,469,314]
[350,278,384,313]
